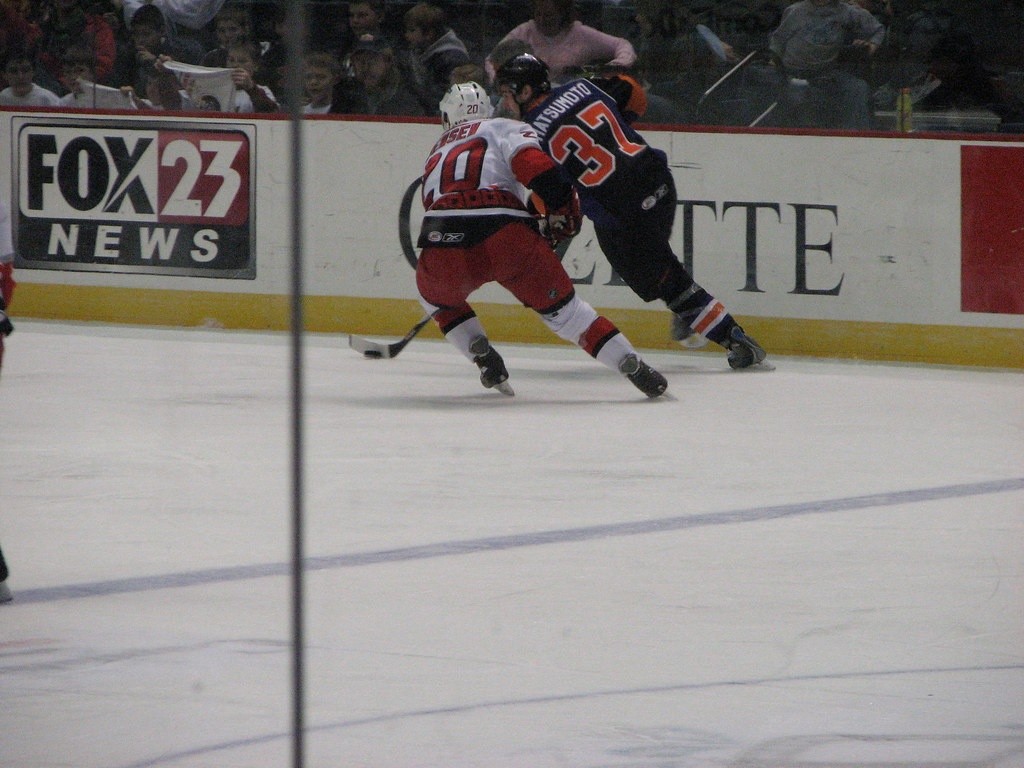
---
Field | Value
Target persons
[0,264,16,582]
[414,81,670,399]
[489,54,765,369]
[0,0,1024,134]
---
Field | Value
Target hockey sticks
[346,219,567,361]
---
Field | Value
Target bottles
[897,88,912,133]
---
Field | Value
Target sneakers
[728,326,775,371]
[618,353,676,401]
[670,312,709,348]
[469,333,514,397]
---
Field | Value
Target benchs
[874,102,1001,129]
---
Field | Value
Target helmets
[493,52,550,91]
[438,81,494,131]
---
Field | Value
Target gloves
[545,189,581,246]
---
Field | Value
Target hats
[350,33,393,58]
